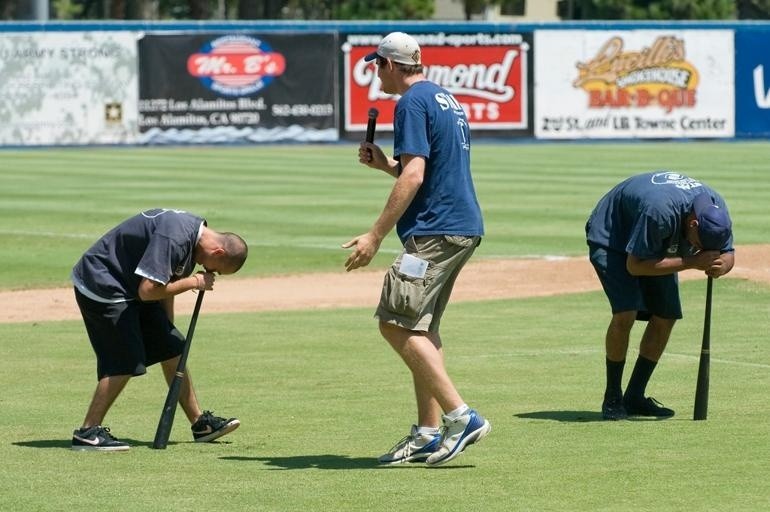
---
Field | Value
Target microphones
[365,108,379,163]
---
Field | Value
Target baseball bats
[694,277,712,421]
[152,290,205,450]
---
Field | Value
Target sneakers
[377,424,443,465]
[426,407,492,466]
[624,397,674,419]
[602,394,628,421]
[71,425,130,452]
[190,410,241,442]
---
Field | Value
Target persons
[70,208,248,450]
[586,170,735,418]
[345,30,490,464]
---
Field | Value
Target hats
[364,31,422,66]
[692,192,732,250]
